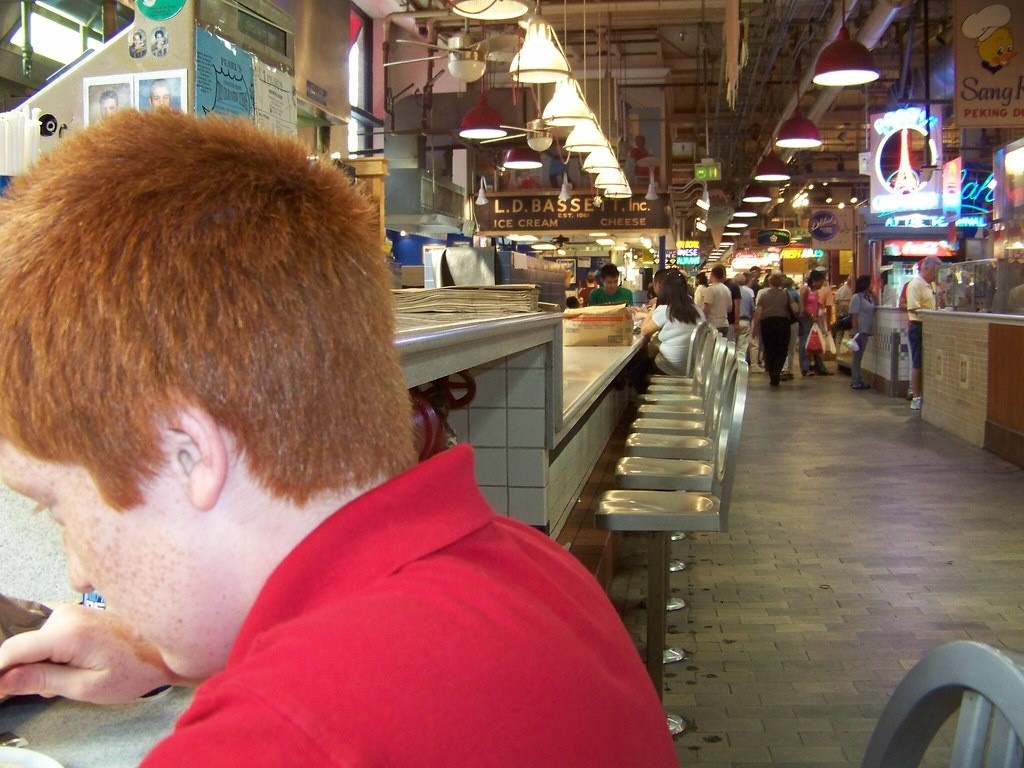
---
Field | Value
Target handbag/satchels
[823,330,836,354]
[785,291,798,324]
[805,323,825,354]
[843,333,859,351]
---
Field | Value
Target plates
[0,746,63,768]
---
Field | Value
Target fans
[383,17,519,82]
[479,82,561,152]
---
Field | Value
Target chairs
[860,640,1024,768]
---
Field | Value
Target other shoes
[817,370,834,376]
[852,381,870,389]
[781,371,794,381]
[802,370,814,375]
[769,381,779,387]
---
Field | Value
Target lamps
[813,0,880,86]
[775,26,823,149]
[558,165,572,202]
[542,66,632,199]
[645,184,659,200]
[458,76,508,140]
[502,91,543,170]
[708,142,792,262]
[509,0,573,83]
[452,0,536,21]
[668,177,710,240]
[531,234,569,250]
[475,176,489,206]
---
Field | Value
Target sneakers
[752,367,765,372]
[910,395,921,410]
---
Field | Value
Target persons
[99,90,118,118]
[564,262,707,393]
[691,266,852,390]
[149,79,172,110]
[130,33,147,58]
[152,30,167,56]
[898,256,945,409]
[848,274,878,389]
[0,107,681,767]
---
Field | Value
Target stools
[594,320,750,735]
